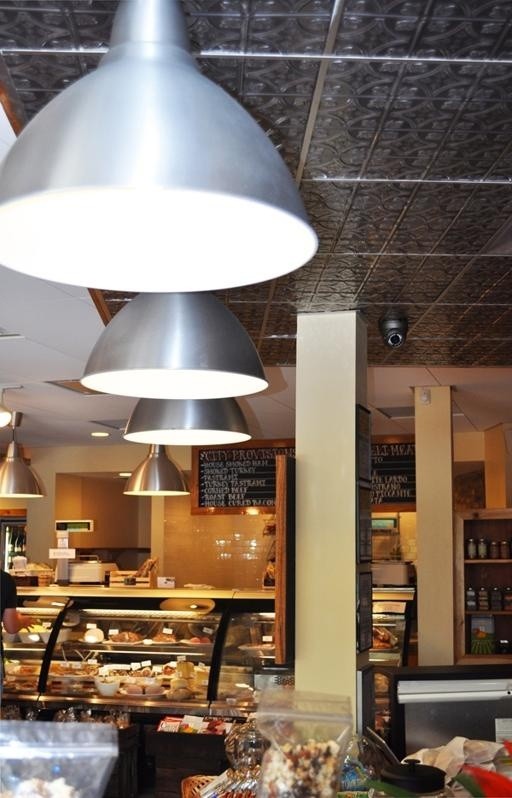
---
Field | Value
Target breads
[163,664,173,675]
[160,598,215,611]
[170,678,189,688]
[374,673,388,693]
[165,688,191,700]
[110,632,139,643]
[375,714,387,731]
[138,681,152,688]
[153,633,175,642]
[127,684,144,693]
[144,684,161,694]
[373,624,399,650]
[23,597,74,609]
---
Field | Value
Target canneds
[501,540,509,559]
[466,539,476,559]
[476,537,488,559]
[490,541,500,559]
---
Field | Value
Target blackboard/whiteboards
[191,433,415,515]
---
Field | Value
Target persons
[0,571,38,679]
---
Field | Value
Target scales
[372,518,416,586]
[53,520,120,587]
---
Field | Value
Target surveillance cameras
[382,316,411,354]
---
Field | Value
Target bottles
[467,588,478,610]
[489,541,498,559]
[491,588,502,611]
[479,538,486,558]
[500,542,509,558]
[503,587,512,611]
[478,588,489,610]
[468,539,476,560]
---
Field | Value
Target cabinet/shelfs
[2,586,275,702]
[452,509,512,666]
[372,587,416,739]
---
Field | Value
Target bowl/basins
[95,678,119,696]
[18,632,41,643]
[40,628,71,643]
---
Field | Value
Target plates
[79,638,100,645]
[103,639,146,646]
[48,672,93,682]
[180,638,215,646]
[145,639,179,646]
[120,688,168,699]
[238,644,276,652]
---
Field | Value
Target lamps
[0,0,319,294]
[1,411,46,498]
[122,399,253,447]
[122,445,190,496]
[78,293,270,399]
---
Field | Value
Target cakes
[111,666,156,678]
[176,660,193,678]
[190,637,200,643]
[194,661,210,701]
[200,637,210,643]
